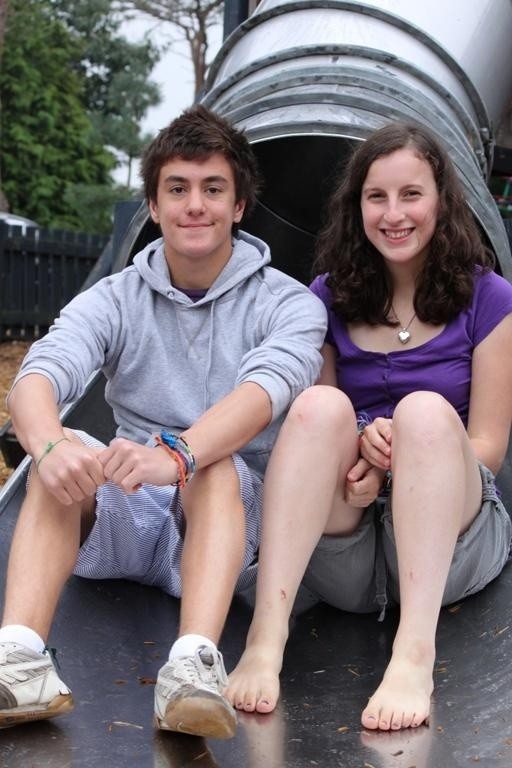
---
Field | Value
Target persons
[221,121,512,731]
[2,104,329,740]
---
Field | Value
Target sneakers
[153,644,238,740]
[0,638,75,730]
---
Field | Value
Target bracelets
[154,430,195,489]
[36,436,71,473]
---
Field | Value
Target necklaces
[389,303,416,343]
